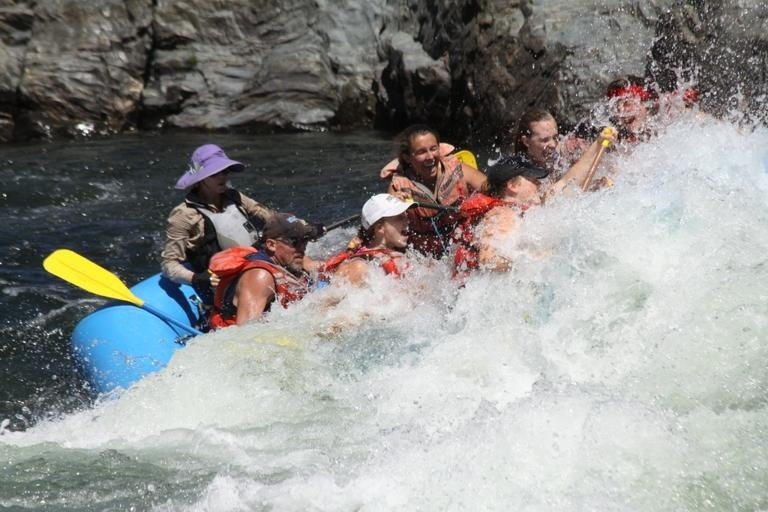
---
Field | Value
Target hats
[487,156,549,189]
[361,193,420,232]
[174,143,245,191]
[262,213,319,244]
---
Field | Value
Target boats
[69,210,330,404]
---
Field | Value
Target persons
[324,81,715,305]
[160,143,324,327]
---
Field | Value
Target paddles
[42,249,204,337]
[326,150,478,230]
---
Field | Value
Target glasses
[209,167,230,178]
[275,234,309,248]
